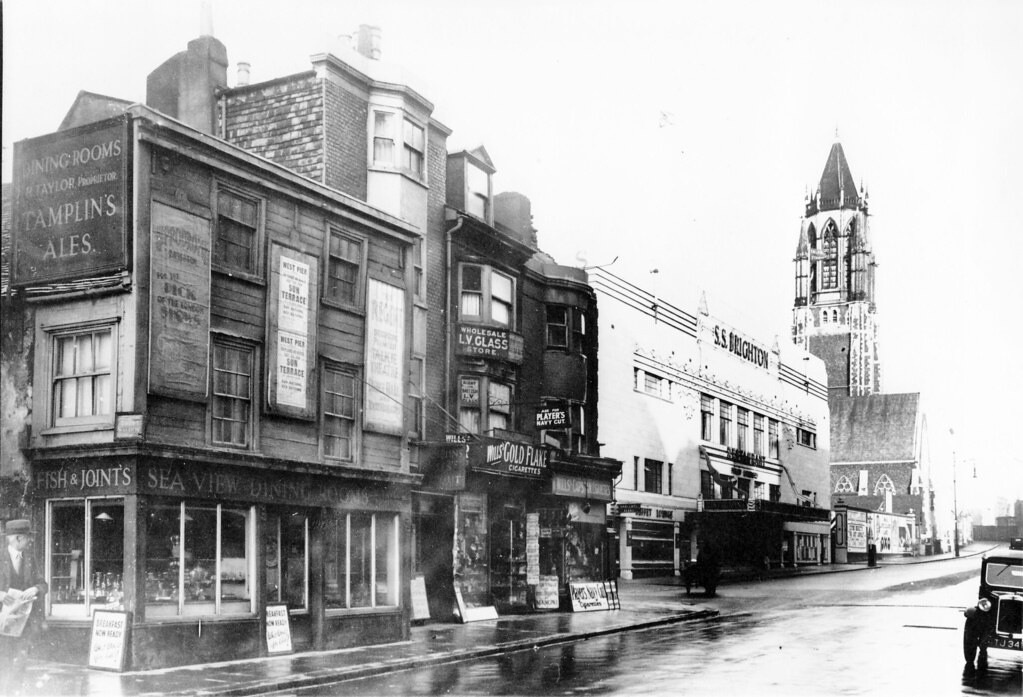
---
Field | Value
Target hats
[1,520,39,536]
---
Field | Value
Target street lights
[953,460,978,556]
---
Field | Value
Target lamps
[95,512,114,522]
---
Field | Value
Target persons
[0,519,48,697]
[697,536,722,599]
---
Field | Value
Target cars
[963,550,1023,663]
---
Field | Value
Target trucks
[1009,536,1022,549]
